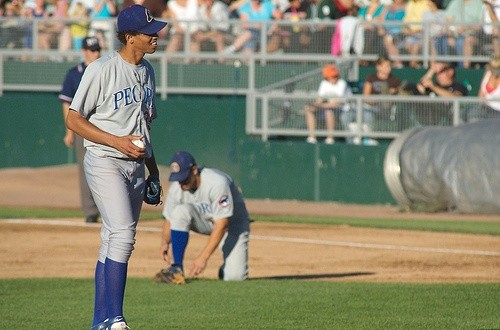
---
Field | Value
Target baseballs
[132,139,144,147]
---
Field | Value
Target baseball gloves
[144,175,163,207]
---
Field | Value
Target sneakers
[155,265,185,284]
[91,315,130,330]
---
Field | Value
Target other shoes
[86,216,98,223]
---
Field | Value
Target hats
[168,151,194,182]
[117,4,167,35]
[82,36,101,51]
[322,64,340,79]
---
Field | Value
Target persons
[153,150,249,284]
[0,0,500,69]
[57,37,101,222]
[66,5,168,330]
[363,56,402,96]
[478,58,500,99]
[419,63,469,97]
[303,64,353,143]
